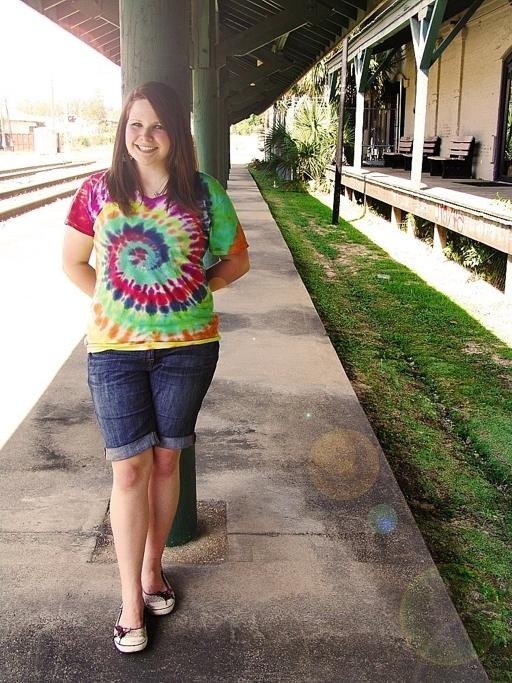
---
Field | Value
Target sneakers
[112,602,149,653]
[141,572,176,616]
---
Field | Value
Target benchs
[381,135,476,179]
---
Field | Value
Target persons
[60,78,250,655]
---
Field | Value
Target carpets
[452,180,512,187]
